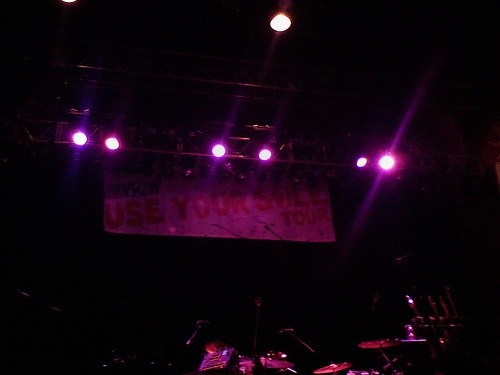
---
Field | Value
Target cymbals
[239,358,295,369]
[205,339,232,353]
[313,362,352,374]
[357,340,400,348]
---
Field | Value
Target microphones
[254,296,262,308]
